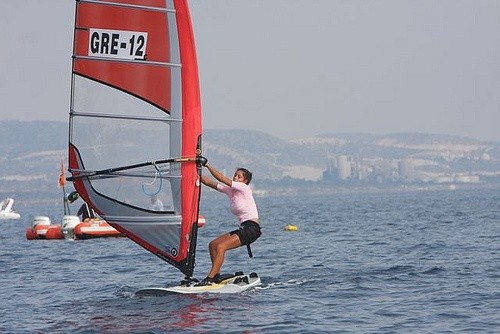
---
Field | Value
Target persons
[146,193,165,212]
[200,161,262,286]
[78,200,98,221]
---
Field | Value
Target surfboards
[134,270,262,296]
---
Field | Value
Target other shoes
[201,273,222,284]
